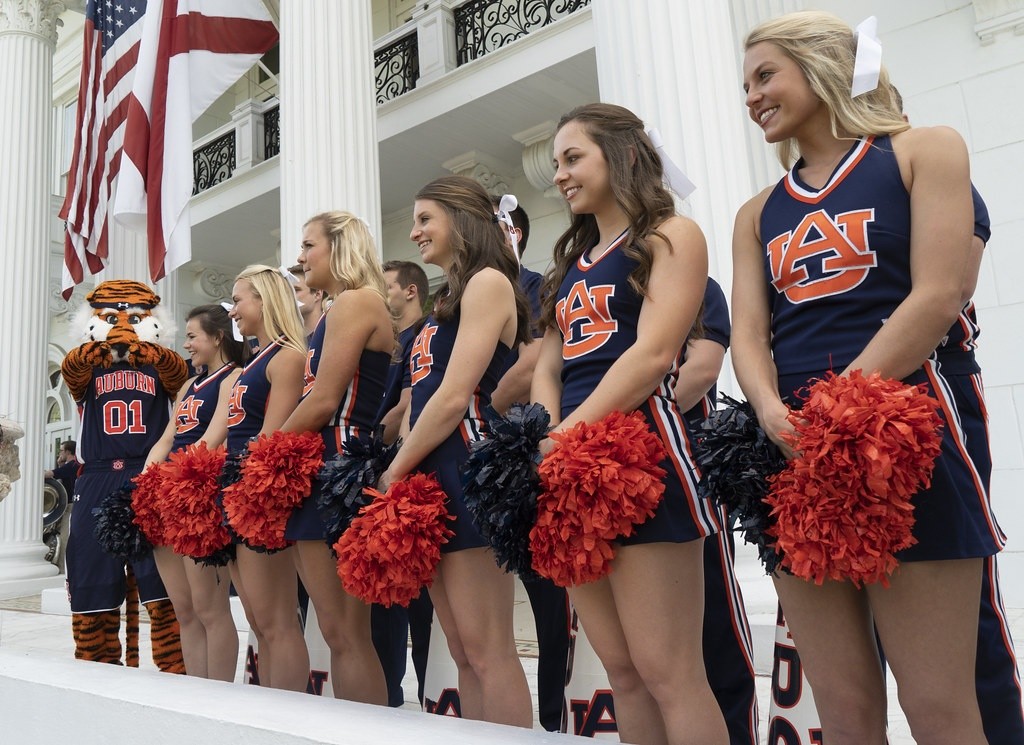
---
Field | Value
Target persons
[730,13,1024,745]
[44,440,80,574]
[530,103,759,745]
[131,175,571,735]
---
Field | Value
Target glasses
[59,449,65,452]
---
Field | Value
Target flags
[112,0,279,284]
[57,0,153,302]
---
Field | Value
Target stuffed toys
[60,280,189,674]
